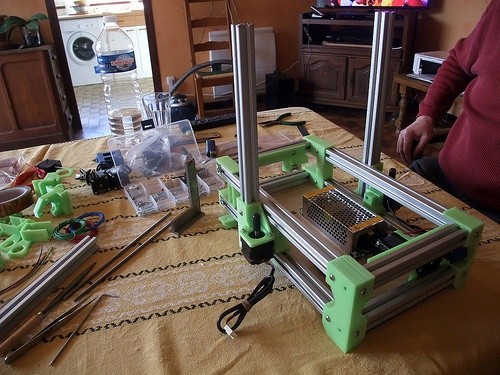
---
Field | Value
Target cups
[141,93,172,128]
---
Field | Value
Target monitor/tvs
[316,0,432,13]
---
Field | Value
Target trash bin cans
[265,71,292,108]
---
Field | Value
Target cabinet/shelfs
[297,9,415,120]
[0,44,74,144]
[110,26,152,81]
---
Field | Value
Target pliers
[258,112,306,128]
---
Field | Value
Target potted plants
[0,10,48,46]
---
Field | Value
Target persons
[396,0,500,226]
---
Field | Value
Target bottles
[92,15,144,140]
[382,168,402,213]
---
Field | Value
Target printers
[407,50,452,83]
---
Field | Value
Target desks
[393,75,463,132]
[0,104,500,375]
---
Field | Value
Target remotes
[179,112,237,133]
